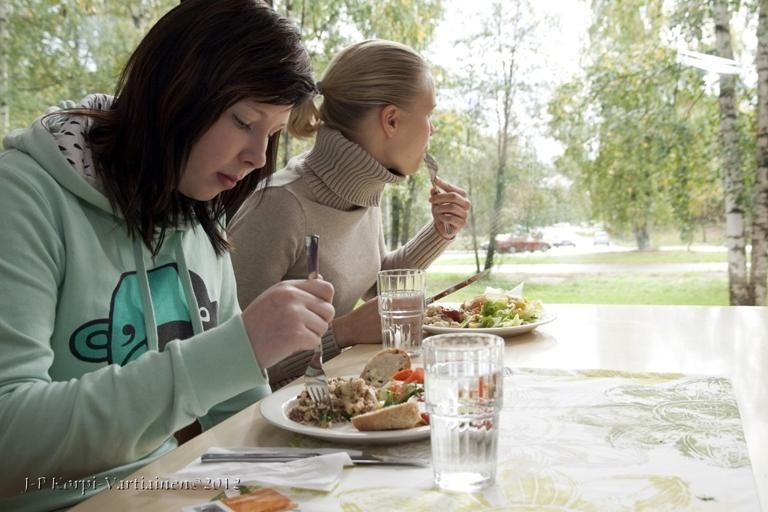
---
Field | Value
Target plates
[420,310,561,338]
[255,377,436,443]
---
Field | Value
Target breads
[352,400,420,431]
[359,348,411,387]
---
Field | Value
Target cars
[593,229,610,246]
[480,225,579,257]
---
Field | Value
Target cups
[422,333,505,493]
[375,269,426,358]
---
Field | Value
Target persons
[225,39,473,394]
[0,0,336,512]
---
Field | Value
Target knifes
[427,266,490,306]
[201,452,429,469]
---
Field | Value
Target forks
[297,231,337,409]
[423,153,454,235]
